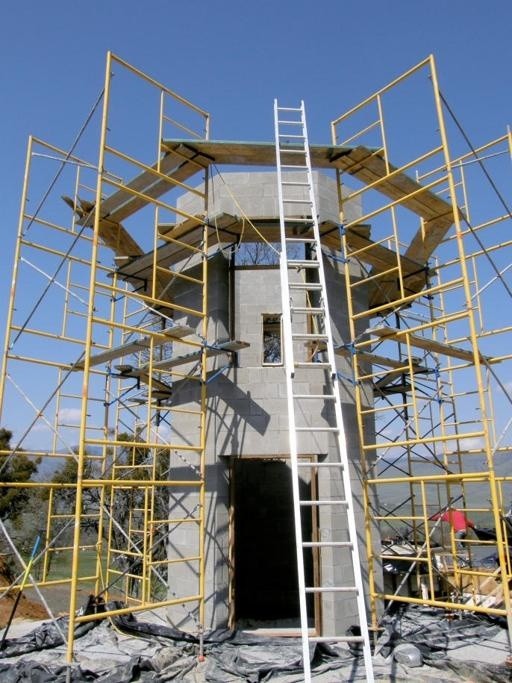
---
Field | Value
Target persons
[429,508,477,569]
